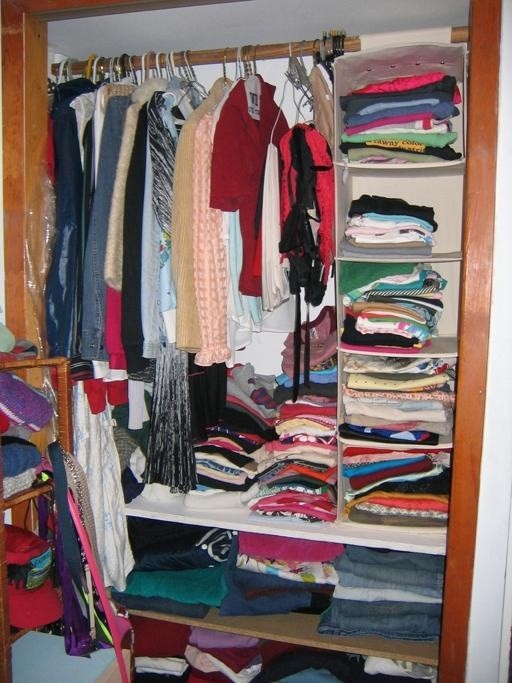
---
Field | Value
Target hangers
[53,28,345,146]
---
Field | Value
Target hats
[4,525,63,629]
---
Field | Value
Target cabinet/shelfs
[0,0,503,683]
